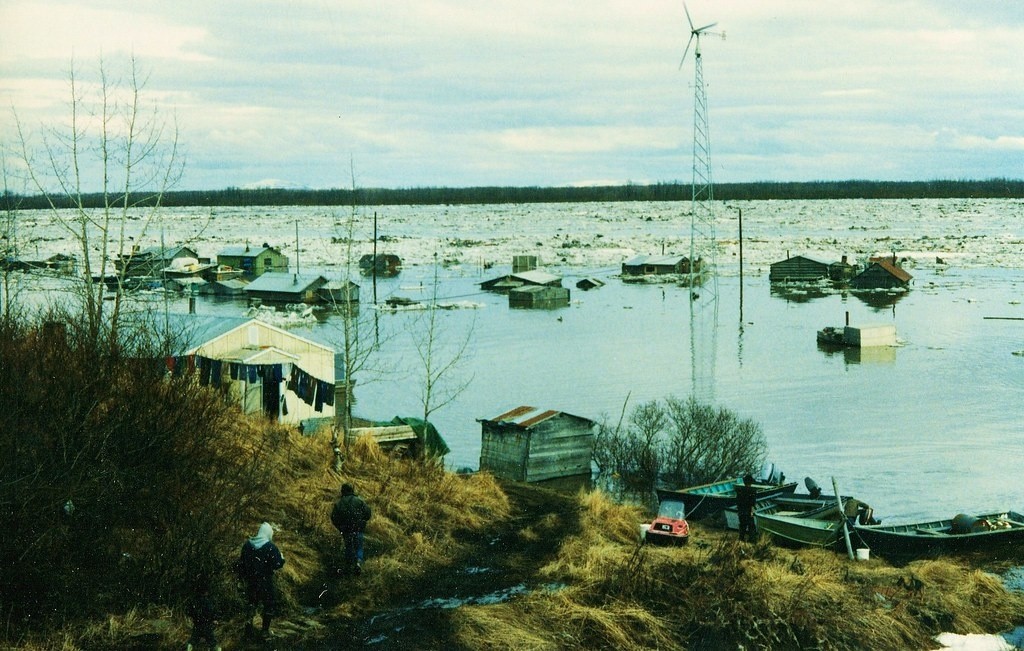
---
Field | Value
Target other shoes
[354,563,362,576]
[346,567,351,574]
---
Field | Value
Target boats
[653,461,800,531]
[755,477,882,553]
[851,510,1024,563]
[755,513,843,550]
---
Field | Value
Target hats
[743,474,755,482]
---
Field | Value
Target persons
[331,482,371,577]
[239,522,285,639]
[185,583,222,651]
[736,474,760,543]
